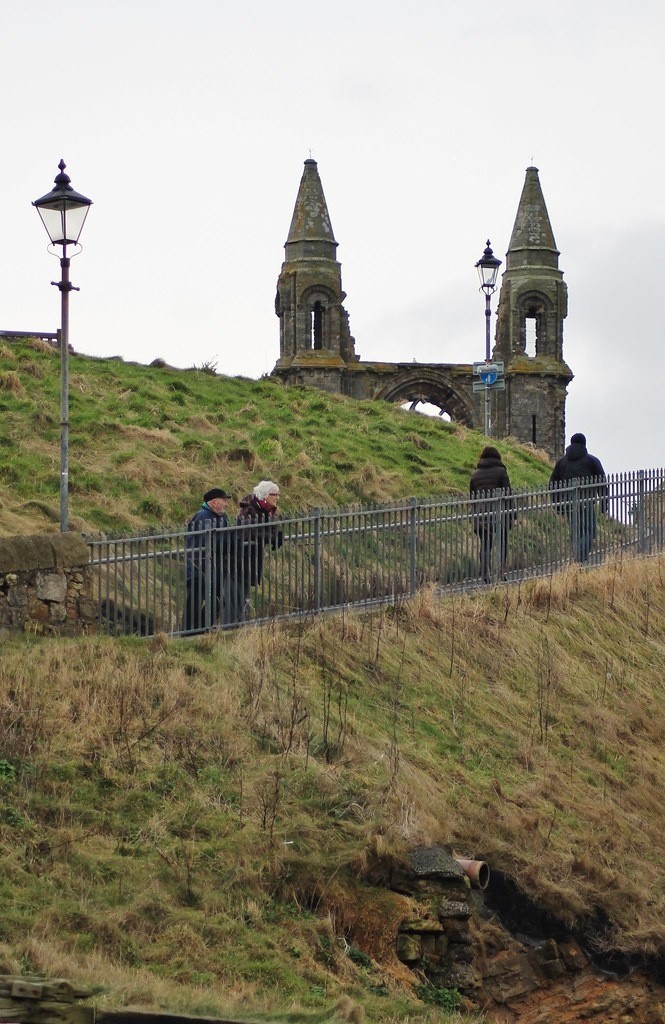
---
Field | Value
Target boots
[498,562,508,582]
[480,552,491,582]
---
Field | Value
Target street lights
[473,238,503,434]
[31,159,96,534]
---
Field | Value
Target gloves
[269,534,282,546]
[272,533,283,550]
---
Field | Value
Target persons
[220,481,285,632]
[549,433,610,564]
[469,444,518,589]
[181,489,238,639]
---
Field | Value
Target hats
[204,488,231,502]
